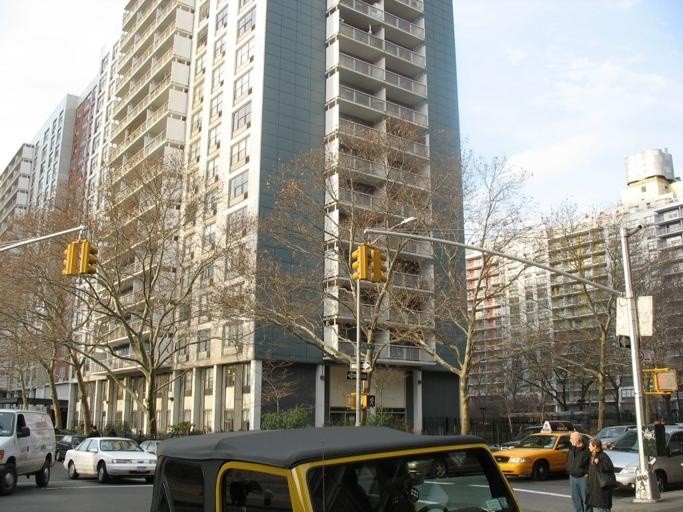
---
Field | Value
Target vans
[0,409,55,494]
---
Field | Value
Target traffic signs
[347,360,372,381]
[619,334,631,349]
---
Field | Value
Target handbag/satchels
[597,472,617,489]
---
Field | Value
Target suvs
[151,422,519,512]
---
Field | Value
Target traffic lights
[61,240,97,275]
[351,245,388,283]
[642,368,679,397]
[345,392,375,410]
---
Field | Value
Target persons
[375,466,415,512]
[583,438,614,512]
[566,431,594,512]
[90,425,100,438]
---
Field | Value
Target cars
[593,425,637,451]
[499,426,543,452]
[496,421,592,482]
[407,451,481,481]
[63,437,158,484]
[55,435,90,462]
[604,423,683,493]
[140,440,161,455]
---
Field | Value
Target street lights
[355,217,417,426]
